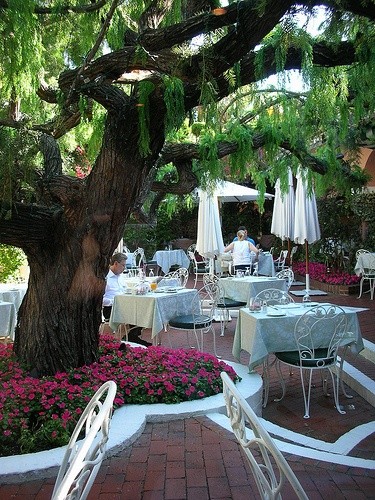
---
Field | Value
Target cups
[249,297,261,311]
[124,282,157,294]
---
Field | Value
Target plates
[266,311,286,316]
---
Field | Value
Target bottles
[263,301,267,313]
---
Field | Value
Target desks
[151,248,190,278]
[234,307,364,419]
[219,277,286,307]
[126,276,180,287]
[214,249,275,278]
[0,288,27,341]
[122,253,137,269]
[109,288,200,354]
[354,251,375,300]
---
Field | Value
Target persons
[102,253,152,346]
[224,230,259,276]
[234,227,256,246]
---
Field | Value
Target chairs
[50,244,375,500]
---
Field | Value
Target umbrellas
[162,177,275,226]
[294,165,321,300]
[271,166,294,265]
[195,168,225,275]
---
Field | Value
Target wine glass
[126,268,144,278]
[149,269,154,277]
[235,267,258,278]
